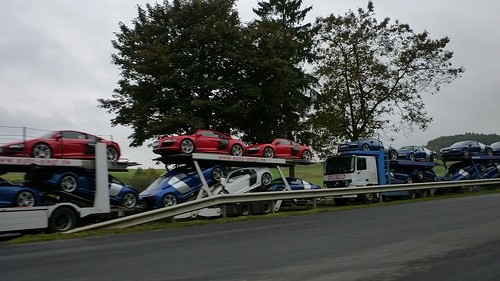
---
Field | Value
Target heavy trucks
[0,122,323,236]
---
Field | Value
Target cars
[210,167,274,196]
[385,161,500,183]
[0,128,121,162]
[440,140,494,158]
[152,129,246,157]
[0,176,43,207]
[138,164,225,209]
[245,136,314,161]
[397,144,439,163]
[262,176,322,202]
[337,138,399,160]
[21,167,139,209]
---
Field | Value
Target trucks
[321,149,500,206]
